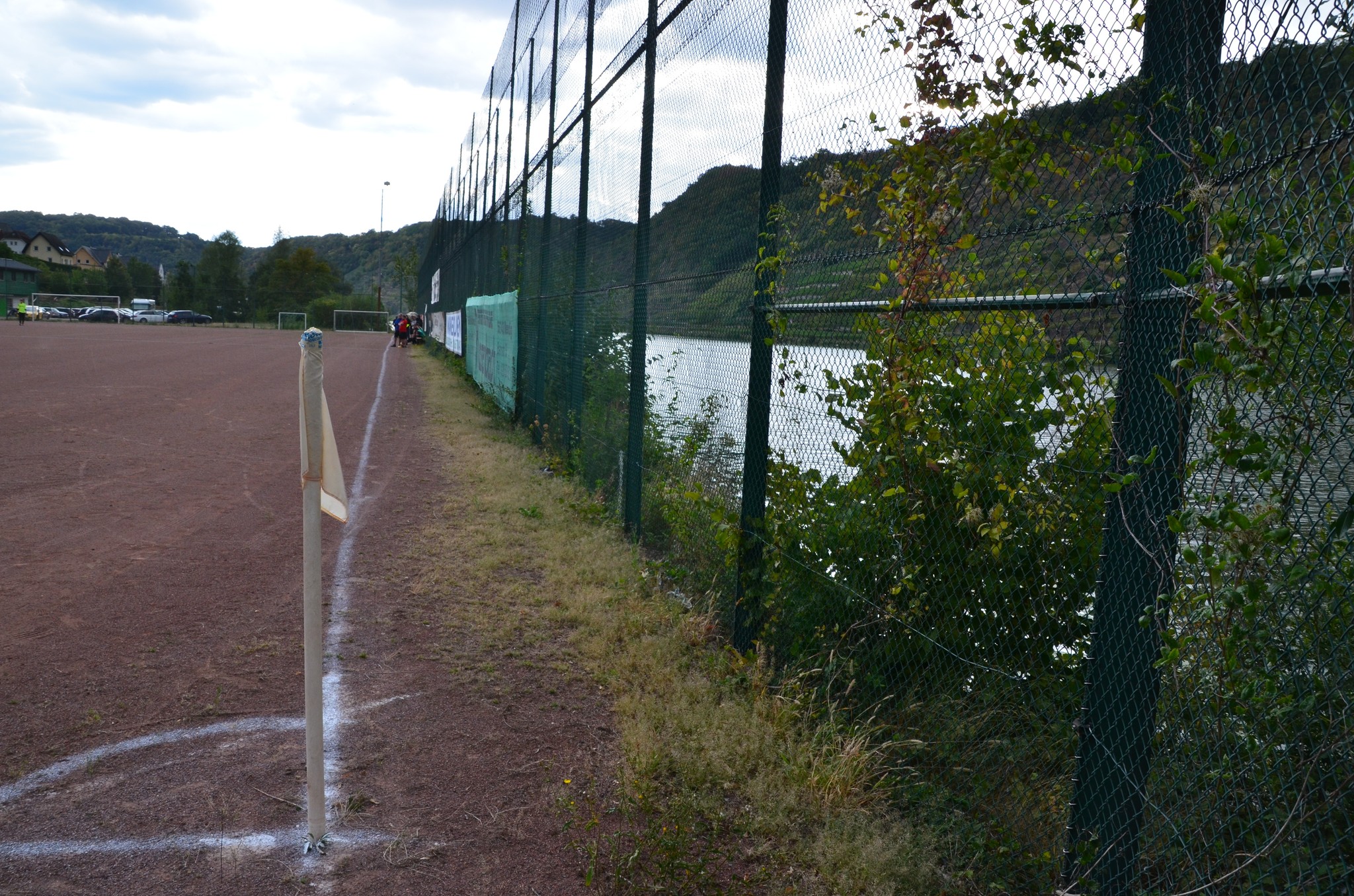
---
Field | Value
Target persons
[16,299,26,326]
[390,313,422,348]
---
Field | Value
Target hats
[20,299,23,301]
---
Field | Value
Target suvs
[167,310,212,325]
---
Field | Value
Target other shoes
[399,344,407,348]
[22,324,24,325]
[391,344,396,347]
[19,324,22,325]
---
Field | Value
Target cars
[17,305,171,324]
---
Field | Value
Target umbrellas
[406,312,418,317]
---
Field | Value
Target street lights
[377,181,389,323]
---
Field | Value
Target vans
[129,298,156,311]
